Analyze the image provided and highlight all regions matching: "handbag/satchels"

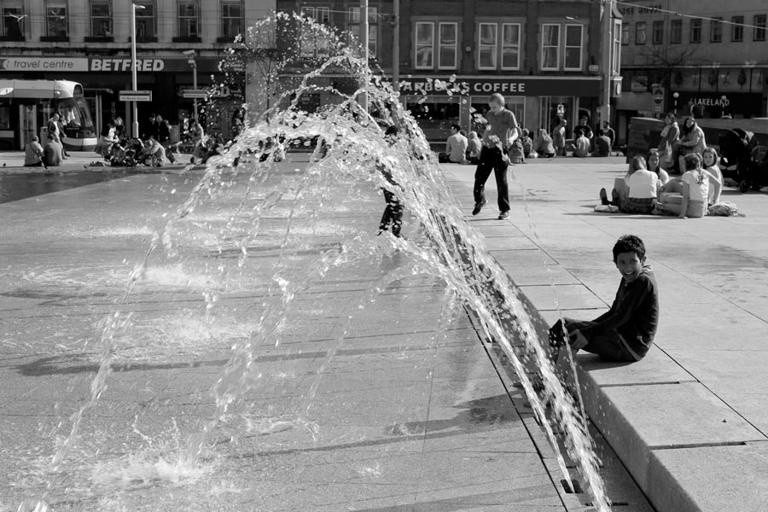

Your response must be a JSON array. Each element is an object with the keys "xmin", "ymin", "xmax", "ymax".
[
  {"xmin": 594, "ymin": 205, "xmax": 618, "ymax": 213},
  {"xmin": 709, "ymin": 201, "xmax": 738, "ymax": 217}
]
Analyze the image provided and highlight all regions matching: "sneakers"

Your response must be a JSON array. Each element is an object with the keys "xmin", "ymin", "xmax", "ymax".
[
  {"xmin": 499, "ymin": 211, "xmax": 510, "ymax": 220},
  {"xmin": 612, "ymin": 188, "xmax": 620, "ymax": 205},
  {"xmin": 600, "ymin": 188, "xmax": 609, "ymax": 205},
  {"xmin": 473, "ymin": 199, "xmax": 487, "ymax": 215}
]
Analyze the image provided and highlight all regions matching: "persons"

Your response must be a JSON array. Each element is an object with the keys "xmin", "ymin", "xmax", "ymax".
[
  {"xmin": 595, "ymin": 110, "xmax": 725, "ymax": 219},
  {"xmin": 472, "ymin": 93, "xmax": 518, "ymax": 222},
  {"xmin": 438, "ymin": 115, "xmax": 594, "ymax": 164},
  {"xmin": 23, "ymin": 112, "xmax": 285, "ymax": 169},
  {"xmin": 514, "ymin": 232, "xmax": 658, "ymax": 407},
  {"xmin": 376, "ymin": 123, "xmax": 416, "ymax": 242}
]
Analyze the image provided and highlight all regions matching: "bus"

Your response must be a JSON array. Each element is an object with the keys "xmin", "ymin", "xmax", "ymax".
[{"xmin": 0, "ymin": 79, "xmax": 97, "ymax": 153}]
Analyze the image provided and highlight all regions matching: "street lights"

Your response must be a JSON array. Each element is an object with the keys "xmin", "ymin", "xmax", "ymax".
[
  {"xmin": 721, "ymin": 95, "xmax": 726, "ymax": 118},
  {"xmin": 185, "ymin": 48, "xmax": 201, "ymax": 126},
  {"xmin": 131, "ymin": 2, "xmax": 148, "ymax": 138},
  {"xmin": 673, "ymin": 92, "xmax": 679, "ymax": 117}
]
